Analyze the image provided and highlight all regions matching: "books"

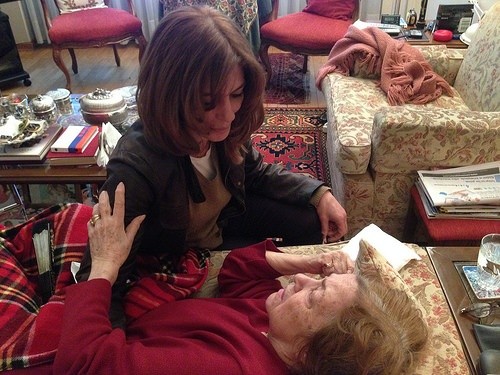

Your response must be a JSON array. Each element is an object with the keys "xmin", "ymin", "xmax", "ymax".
[
  {"xmin": 0, "ymin": 125, "xmax": 103, "ymax": 166},
  {"xmin": 400, "ymin": 29, "xmax": 430, "ymax": 42},
  {"xmin": 415, "ymin": 178, "xmax": 500, "ymax": 222}
]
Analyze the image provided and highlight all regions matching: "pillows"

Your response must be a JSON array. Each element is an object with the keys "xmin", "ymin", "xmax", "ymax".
[
  {"xmin": 56, "ymin": 0, "xmax": 109, "ymax": 14},
  {"xmin": 302, "ymin": 0, "xmax": 357, "ymax": 21}
]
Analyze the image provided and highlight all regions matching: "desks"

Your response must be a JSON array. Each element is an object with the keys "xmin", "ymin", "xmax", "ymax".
[
  {"xmin": 402, "ymin": 186, "xmax": 500, "ymax": 247},
  {"xmin": 426, "ymin": 247, "xmax": 500, "ymax": 375}
]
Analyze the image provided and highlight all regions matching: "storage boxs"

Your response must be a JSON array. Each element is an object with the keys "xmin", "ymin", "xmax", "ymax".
[{"xmin": 436, "ymin": 5, "xmax": 474, "ymax": 34}]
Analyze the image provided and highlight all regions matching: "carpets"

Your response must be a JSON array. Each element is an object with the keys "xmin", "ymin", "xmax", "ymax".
[
  {"xmin": 258, "ymin": 53, "xmax": 311, "ymax": 104},
  {"xmin": 252, "ymin": 106, "xmax": 345, "ymax": 245}
]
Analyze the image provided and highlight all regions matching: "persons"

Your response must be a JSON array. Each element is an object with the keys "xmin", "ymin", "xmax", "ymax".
[
  {"xmin": 65, "ymin": 6, "xmax": 349, "ymax": 268},
  {"xmin": 0, "ymin": 179, "xmax": 427, "ymax": 375}
]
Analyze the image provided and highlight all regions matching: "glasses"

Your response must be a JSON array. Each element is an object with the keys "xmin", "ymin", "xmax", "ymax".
[{"xmin": 460, "ymin": 300, "xmax": 500, "ymax": 318}]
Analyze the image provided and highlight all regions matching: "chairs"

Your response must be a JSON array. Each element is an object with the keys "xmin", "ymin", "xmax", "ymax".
[
  {"xmin": 258, "ymin": 0, "xmax": 360, "ymax": 92},
  {"xmin": 40, "ymin": 0, "xmax": 147, "ymax": 94}
]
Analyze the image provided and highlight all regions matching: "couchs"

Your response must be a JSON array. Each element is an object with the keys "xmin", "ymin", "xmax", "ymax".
[
  {"xmin": 196, "ymin": 244, "xmax": 472, "ymax": 375},
  {"xmin": 320, "ymin": 2, "xmax": 500, "ymax": 247}
]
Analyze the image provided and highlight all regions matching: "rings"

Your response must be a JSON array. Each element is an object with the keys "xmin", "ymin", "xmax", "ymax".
[
  {"xmin": 90, "ymin": 215, "xmax": 100, "ymax": 226},
  {"xmin": 324, "ymin": 262, "xmax": 334, "ymax": 268}
]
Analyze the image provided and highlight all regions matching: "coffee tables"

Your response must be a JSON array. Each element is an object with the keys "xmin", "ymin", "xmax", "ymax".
[{"xmin": 0, "ymin": 95, "xmax": 127, "ymax": 204}]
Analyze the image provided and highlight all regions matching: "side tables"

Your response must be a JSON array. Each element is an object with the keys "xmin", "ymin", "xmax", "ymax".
[{"xmin": 364, "ymin": 18, "xmax": 468, "ymax": 48}]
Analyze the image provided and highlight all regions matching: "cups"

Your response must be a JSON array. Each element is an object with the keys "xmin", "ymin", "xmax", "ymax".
[
  {"xmin": 7, "ymin": 93, "xmax": 28, "ymax": 120},
  {"xmin": 54, "ymin": 88, "xmax": 74, "ymax": 116},
  {"xmin": 476, "ymin": 234, "xmax": 500, "ymax": 292}
]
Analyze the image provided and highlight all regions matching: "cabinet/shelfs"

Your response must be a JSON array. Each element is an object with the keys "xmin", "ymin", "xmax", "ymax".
[{"xmin": 0, "ymin": 11, "xmax": 31, "ymax": 86}]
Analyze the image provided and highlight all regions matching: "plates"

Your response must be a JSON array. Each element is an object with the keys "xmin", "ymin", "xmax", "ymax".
[{"xmin": 0, "ymin": 121, "xmax": 48, "ymax": 146}]
